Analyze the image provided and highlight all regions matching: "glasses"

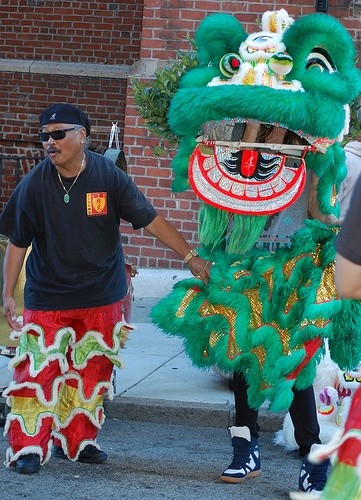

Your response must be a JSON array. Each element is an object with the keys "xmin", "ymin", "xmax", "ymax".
[{"xmin": 38, "ymin": 126, "xmax": 83, "ymax": 142}]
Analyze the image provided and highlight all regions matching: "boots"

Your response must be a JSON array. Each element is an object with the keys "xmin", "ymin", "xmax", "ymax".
[
  {"xmin": 299, "ymin": 457, "xmax": 332, "ymax": 492},
  {"xmin": 221, "ymin": 425, "xmax": 262, "ymax": 483}
]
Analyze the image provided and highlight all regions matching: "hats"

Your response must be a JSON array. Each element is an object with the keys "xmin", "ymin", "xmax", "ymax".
[{"xmin": 39, "ymin": 104, "xmax": 90, "ymax": 137}]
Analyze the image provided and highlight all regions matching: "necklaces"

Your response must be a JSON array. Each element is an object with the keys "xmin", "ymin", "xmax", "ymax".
[{"xmin": 49, "ymin": 151, "xmax": 85, "ymax": 204}]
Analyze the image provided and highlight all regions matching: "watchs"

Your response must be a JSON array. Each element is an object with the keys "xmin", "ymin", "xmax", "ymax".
[{"xmin": 183, "ymin": 249, "xmax": 198, "ymax": 263}]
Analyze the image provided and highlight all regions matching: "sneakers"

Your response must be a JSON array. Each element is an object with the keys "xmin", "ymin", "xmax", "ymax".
[
  {"xmin": 16, "ymin": 453, "xmax": 40, "ymax": 473},
  {"xmin": 54, "ymin": 443, "xmax": 107, "ymax": 463}
]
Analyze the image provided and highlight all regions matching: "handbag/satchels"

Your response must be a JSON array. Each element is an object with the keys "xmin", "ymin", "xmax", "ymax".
[{"xmin": 103, "ymin": 122, "xmax": 128, "ymax": 175}]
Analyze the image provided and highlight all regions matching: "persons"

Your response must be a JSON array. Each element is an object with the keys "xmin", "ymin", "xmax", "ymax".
[
  {"xmin": 1, "ymin": 104, "xmax": 215, "ymax": 468},
  {"xmin": 220, "ymin": 120, "xmax": 331, "ymax": 492},
  {"xmin": 333, "ymin": 170, "xmax": 361, "ymax": 300}
]
